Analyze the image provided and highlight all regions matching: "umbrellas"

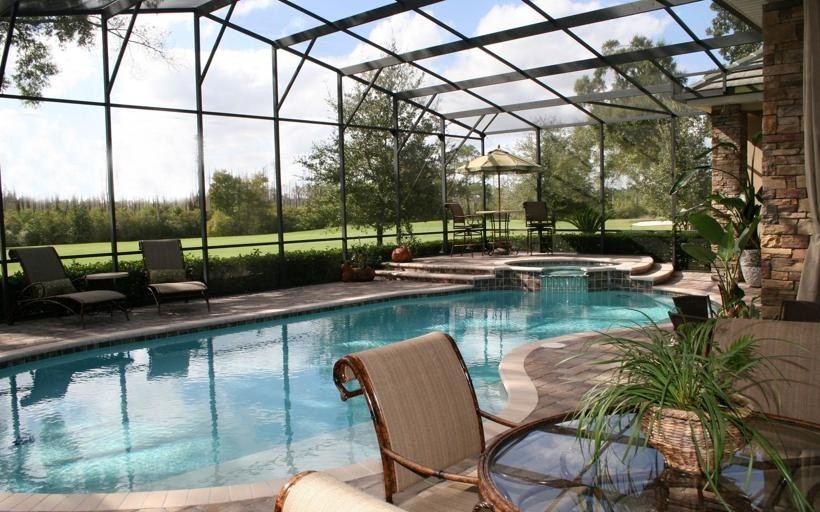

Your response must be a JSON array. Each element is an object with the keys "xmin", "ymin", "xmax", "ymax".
[{"xmin": 456, "ymin": 144, "xmax": 542, "ymax": 236}]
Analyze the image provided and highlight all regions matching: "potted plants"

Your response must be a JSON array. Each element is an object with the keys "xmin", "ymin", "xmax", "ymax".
[
  {"xmin": 667, "ymin": 128, "xmax": 764, "ymax": 287},
  {"xmin": 342, "ymin": 242, "xmax": 383, "ymax": 282},
  {"xmin": 568, "ymin": 304, "xmax": 820, "ymax": 512}
]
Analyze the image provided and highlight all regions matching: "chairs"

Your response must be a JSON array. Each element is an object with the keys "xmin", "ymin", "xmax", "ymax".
[
  {"xmin": 522, "ymin": 201, "xmax": 556, "ymax": 256},
  {"xmin": 8, "ymin": 238, "xmax": 212, "ymax": 329},
  {"xmin": 275, "ymin": 466, "xmax": 414, "ymax": 512},
  {"xmin": 330, "ymin": 334, "xmax": 523, "ymax": 512},
  {"xmin": 445, "ymin": 202, "xmax": 484, "ymax": 258}
]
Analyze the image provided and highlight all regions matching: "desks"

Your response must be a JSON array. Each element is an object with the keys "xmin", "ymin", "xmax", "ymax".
[
  {"xmin": 476, "ymin": 402, "xmax": 818, "ymax": 512},
  {"xmin": 475, "ymin": 209, "xmax": 524, "ymax": 256}
]
